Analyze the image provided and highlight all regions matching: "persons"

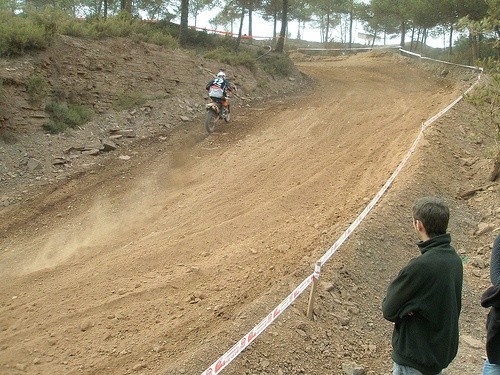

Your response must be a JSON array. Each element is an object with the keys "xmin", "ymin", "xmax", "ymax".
[
  {"xmin": 380, "ymin": 196, "xmax": 464, "ymax": 375},
  {"xmin": 205, "ymin": 71, "xmax": 236, "ymax": 120},
  {"xmin": 480, "ymin": 234, "xmax": 499, "ymax": 375}
]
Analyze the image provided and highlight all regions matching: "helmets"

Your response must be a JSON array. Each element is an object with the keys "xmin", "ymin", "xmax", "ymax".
[{"xmin": 216, "ymin": 71, "xmax": 226, "ymax": 77}]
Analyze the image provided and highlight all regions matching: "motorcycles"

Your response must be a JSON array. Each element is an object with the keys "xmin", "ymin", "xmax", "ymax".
[{"xmin": 205, "ymin": 87, "xmax": 231, "ymax": 133}]
